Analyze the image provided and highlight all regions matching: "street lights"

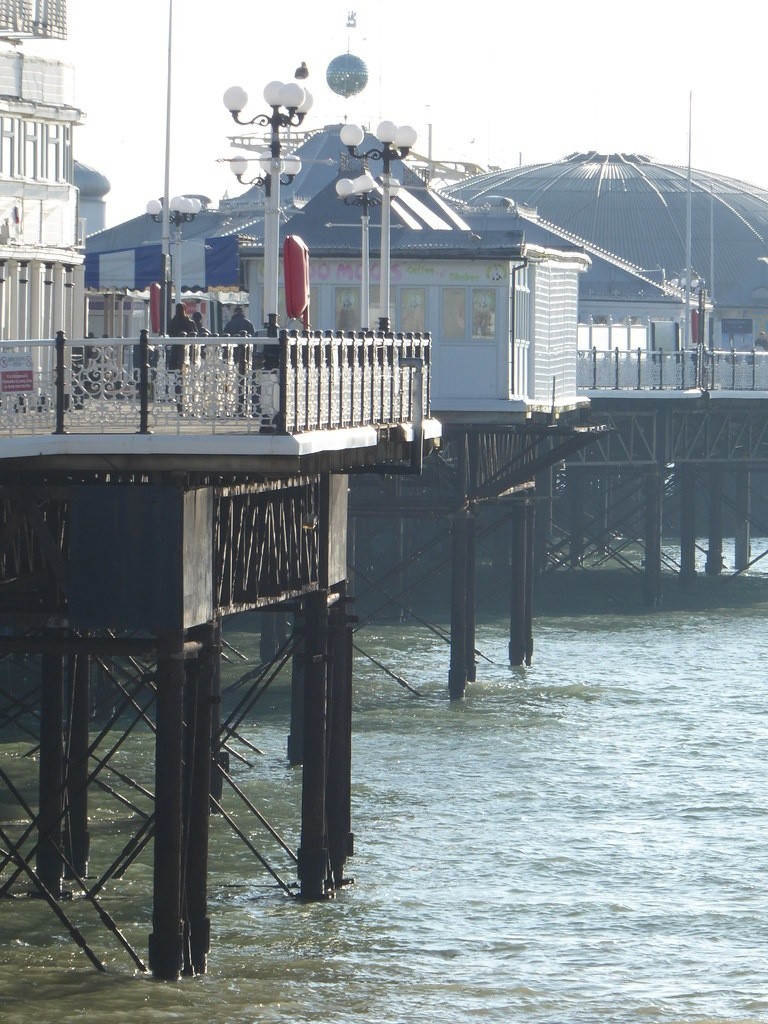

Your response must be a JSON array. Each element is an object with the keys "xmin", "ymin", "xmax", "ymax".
[
  {"xmin": 336, "ymin": 175, "xmax": 401, "ymax": 332},
  {"xmin": 214, "ymin": 80, "xmax": 313, "ymax": 434},
  {"xmin": 146, "ymin": 196, "xmax": 202, "ymax": 305},
  {"xmin": 670, "ymin": 277, "xmax": 699, "ymax": 361},
  {"xmin": 340, "ymin": 120, "xmax": 418, "ymax": 332},
  {"xmin": 231, "ymin": 152, "xmax": 302, "ymax": 336}
]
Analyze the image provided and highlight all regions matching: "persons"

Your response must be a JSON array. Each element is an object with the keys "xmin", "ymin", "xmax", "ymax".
[
  {"xmin": 192, "ymin": 306, "xmax": 255, "ymax": 365},
  {"xmin": 168, "ymin": 303, "xmax": 197, "ymax": 413},
  {"xmin": 755, "ymin": 332, "xmax": 767, "ymax": 350}
]
